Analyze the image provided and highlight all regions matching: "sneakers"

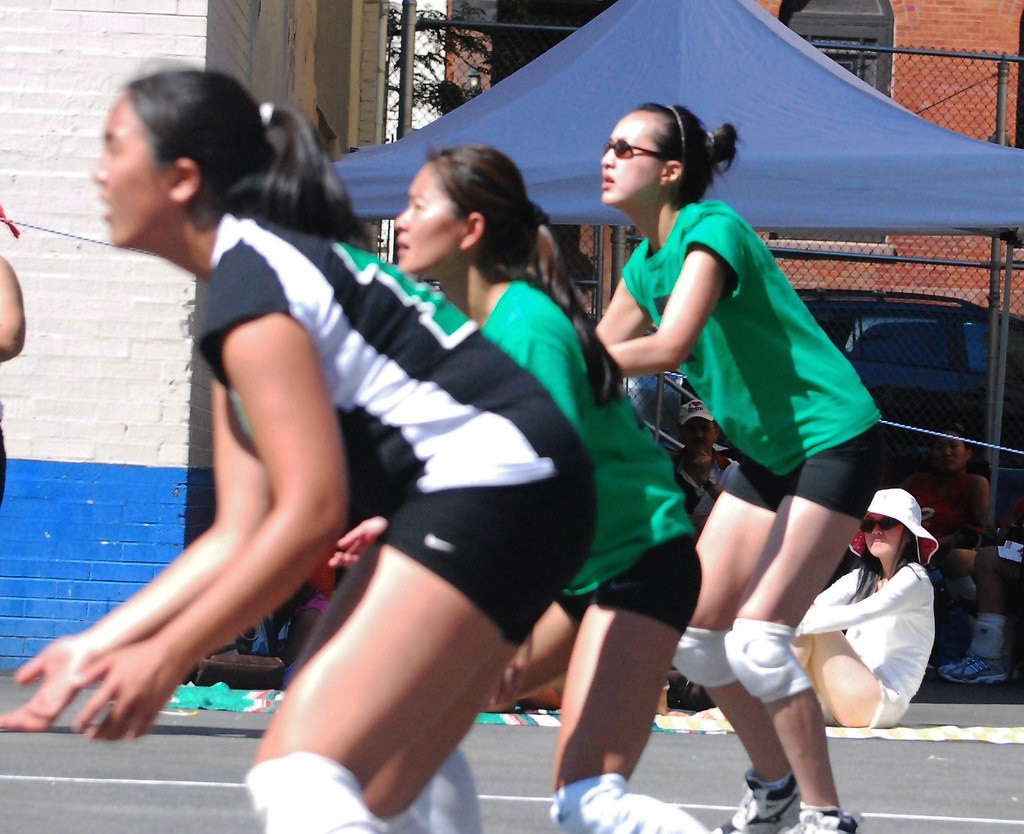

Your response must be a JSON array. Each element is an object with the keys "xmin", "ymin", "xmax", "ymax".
[
  {"xmin": 712, "ymin": 772, "xmax": 800, "ymax": 834},
  {"xmin": 783, "ymin": 802, "xmax": 858, "ymax": 834},
  {"xmin": 938, "ymin": 655, "xmax": 1009, "ymax": 684}
]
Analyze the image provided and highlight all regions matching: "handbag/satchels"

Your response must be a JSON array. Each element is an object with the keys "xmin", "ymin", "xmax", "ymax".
[{"xmin": 667, "ymin": 672, "xmax": 708, "ymax": 712}]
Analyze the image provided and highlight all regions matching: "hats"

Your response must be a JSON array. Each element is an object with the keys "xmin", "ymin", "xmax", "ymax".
[
  {"xmin": 848, "ymin": 488, "xmax": 939, "ymax": 566},
  {"xmin": 679, "ymin": 399, "xmax": 714, "ymax": 425}
]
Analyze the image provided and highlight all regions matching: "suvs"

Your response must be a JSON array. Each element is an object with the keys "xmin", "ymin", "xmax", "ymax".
[{"xmin": 793, "ymin": 288, "xmax": 1024, "ymax": 470}]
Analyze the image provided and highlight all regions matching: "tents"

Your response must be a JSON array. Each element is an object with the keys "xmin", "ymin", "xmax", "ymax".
[{"xmin": 330, "ymin": 0, "xmax": 1024, "ymax": 521}]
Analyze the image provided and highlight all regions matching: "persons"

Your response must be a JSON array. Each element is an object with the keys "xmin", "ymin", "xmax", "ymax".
[
  {"xmin": 0, "ymin": 255, "xmax": 26, "ymax": 506},
  {"xmin": 938, "ymin": 499, "xmax": 1024, "ymax": 683},
  {"xmin": 790, "ymin": 487, "xmax": 937, "ymax": 729},
  {"xmin": 897, "ymin": 425, "xmax": 990, "ymax": 595},
  {"xmin": 0, "ymin": 69, "xmax": 598, "ymax": 834},
  {"xmin": 399, "ymin": 143, "xmax": 713, "ymax": 834},
  {"xmin": 664, "ymin": 399, "xmax": 732, "ymax": 515},
  {"xmin": 599, "ymin": 103, "xmax": 882, "ymax": 834}
]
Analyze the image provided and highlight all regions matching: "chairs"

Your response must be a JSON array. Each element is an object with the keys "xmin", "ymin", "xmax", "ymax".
[{"xmin": 995, "ymin": 465, "xmax": 1024, "ymax": 530}]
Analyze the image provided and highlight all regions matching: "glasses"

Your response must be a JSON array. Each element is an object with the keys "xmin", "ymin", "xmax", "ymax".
[
  {"xmin": 603, "ymin": 139, "xmax": 670, "ymax": 161},
  {"xmin": 860, "ymin": 517, "xmax": 901, "ymax": 532}
]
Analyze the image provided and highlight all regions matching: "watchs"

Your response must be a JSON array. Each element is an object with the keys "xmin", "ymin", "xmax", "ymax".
[{"xmin": 704, "ymin": 479, "xmax": 718, "ymax": 490}]
